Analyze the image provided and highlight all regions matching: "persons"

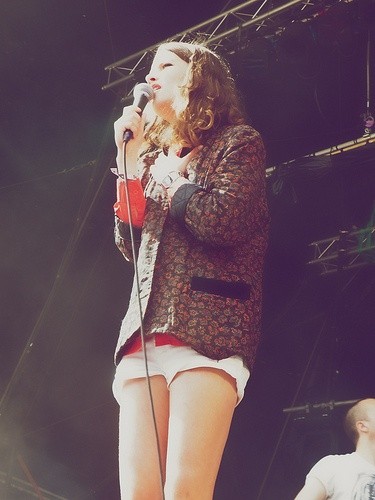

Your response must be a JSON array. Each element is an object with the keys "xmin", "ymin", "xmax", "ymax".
[
  {"xmin": 294, "ymin": 396, "xmax": 374, "ymax": 500},
  {"xmin": 110, "ymin": 40, "xmax": 270, "ymax": 500}
]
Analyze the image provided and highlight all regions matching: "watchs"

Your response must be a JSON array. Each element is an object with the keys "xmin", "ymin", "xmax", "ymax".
[{"xmin": 162, "ymin": 170, "xmax": 184, "ymax": 189}]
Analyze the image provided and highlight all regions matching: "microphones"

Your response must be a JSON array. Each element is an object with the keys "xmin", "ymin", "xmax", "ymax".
[{"xmin": 123, "ymin": 83, "xmax": 154, "ymax": 142}]
